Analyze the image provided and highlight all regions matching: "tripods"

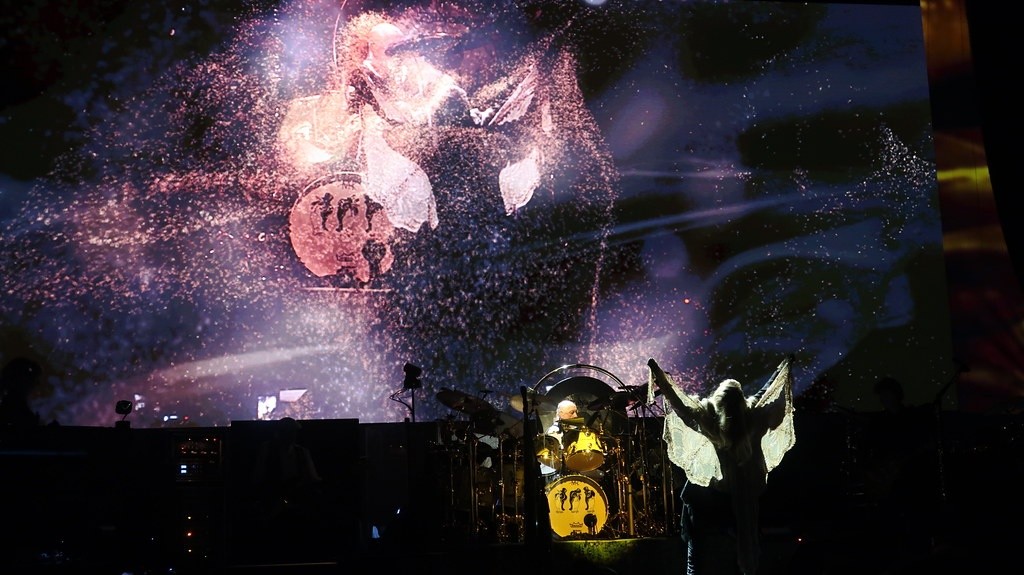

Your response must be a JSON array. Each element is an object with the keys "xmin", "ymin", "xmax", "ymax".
[{"xmin": 608, "ymin": 401, "xmax": 665, "ymax": 539}]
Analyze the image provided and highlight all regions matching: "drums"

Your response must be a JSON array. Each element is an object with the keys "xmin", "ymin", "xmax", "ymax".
[
  {"xmin": 533, "ymin": 434, "xmax": 561, "ymax": 477},
  {"xmin": 542, "ymin": 473, "xmax": 611, "ymax": 542},
  {"xmin": 561, "ymin": 427, "xmax": 606, "ymax": 474}
]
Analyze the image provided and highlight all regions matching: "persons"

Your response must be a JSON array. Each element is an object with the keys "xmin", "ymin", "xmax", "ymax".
[
  {"xmin": 646, "ymin": 357, "xmax": 798, "ymax": 575},
  {"xmin": 541, "ymin": 401, "xmax": 586, "ymax": 450}
]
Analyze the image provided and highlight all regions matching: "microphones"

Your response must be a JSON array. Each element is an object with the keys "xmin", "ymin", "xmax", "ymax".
[{"xmin": 481, "ymin": 390, "xmax": 493, "ymax": 393}]
